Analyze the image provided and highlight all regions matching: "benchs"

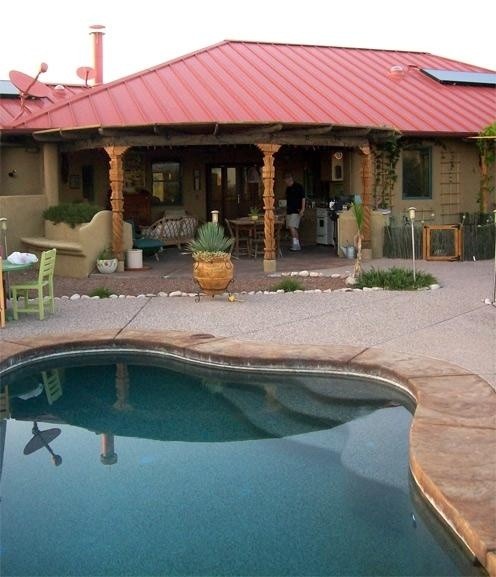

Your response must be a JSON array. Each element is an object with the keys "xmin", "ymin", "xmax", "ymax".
[{"xmin": 138, "ymin": 215, "xmax": 200, "ymax": 262}]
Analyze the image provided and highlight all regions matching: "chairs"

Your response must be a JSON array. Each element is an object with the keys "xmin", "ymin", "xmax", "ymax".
[
  {"xmin": 225, "ymin": 214, "xmax": 285, "ymax": 258},
  {"xmin": 11, "ymin": 247, "xmax": 57, "ymax": 321}
]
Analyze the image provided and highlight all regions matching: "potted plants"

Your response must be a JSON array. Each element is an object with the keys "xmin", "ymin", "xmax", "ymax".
[
  {"xmin": 181, "ymin": 222, "xmax": 238, "ymax": 295},
  {"xmin": 248, "ymin": 206, "xmax": 259, "ymax": 220},
  {"xmin": 94, "ymin": 246, "xmax": 118, "ymax": 273}
]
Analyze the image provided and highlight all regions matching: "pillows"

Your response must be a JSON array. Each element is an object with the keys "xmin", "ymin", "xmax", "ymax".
[{"xmin": 151, "ymin": 218, "xmax": 196, "ymax": 239}]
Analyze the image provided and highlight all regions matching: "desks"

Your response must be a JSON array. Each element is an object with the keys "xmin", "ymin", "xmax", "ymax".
[{"xmin": 1, "ymin": 259, "xmax": 34, "ymax": 323}]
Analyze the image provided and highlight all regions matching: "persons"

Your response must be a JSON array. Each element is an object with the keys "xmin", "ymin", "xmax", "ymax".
[{"xmin": 281, "ymin": 172, "xmax": 306, "ymax": 251}]
